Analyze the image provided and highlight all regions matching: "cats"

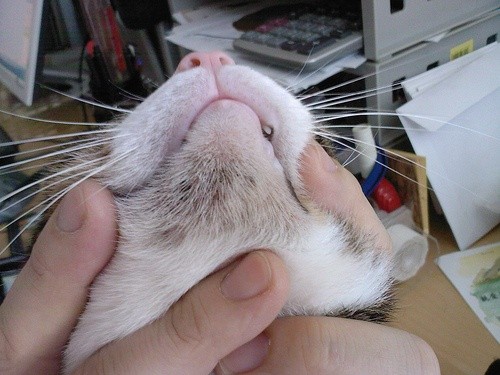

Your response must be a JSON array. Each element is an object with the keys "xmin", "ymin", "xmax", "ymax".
[{"xmin": 2, "ymin": 45, "xmax": 498, "ymax": 370}]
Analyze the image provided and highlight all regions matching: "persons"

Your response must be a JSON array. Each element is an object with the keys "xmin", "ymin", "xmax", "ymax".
[{"xmin": 1, "ymin": 178, "xmax": 442, "ymax": 373}]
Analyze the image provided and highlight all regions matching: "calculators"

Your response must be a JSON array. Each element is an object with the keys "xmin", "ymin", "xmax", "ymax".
[{"xmin": 232, "ymin": 2, "xmax": 364, "ymax": 72}]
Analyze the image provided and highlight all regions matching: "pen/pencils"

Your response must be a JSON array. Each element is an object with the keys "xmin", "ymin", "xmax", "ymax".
[{"xmin": 83, "ymin": 35, "xmax": 142, "ymax": 94}]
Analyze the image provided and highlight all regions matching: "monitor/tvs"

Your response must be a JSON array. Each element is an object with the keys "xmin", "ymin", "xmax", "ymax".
[{"xmin": 0, "ymin": 0, "xmax": 45, "ymax": 106}]
